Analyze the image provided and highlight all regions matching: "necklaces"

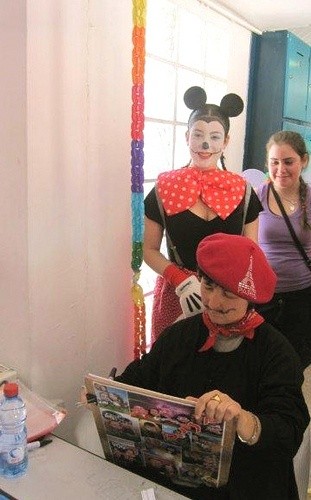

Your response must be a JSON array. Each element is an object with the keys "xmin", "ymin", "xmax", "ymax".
[{"xmin": 280, "ymin": 195, "xmax": 298, "ymax": 212}]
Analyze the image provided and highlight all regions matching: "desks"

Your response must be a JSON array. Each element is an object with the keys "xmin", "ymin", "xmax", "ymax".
[{"xmin": 0, "ymin": 433, "xmax": 191, "ymax": 500}]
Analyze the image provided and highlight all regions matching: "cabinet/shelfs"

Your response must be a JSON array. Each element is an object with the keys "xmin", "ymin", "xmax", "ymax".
[{"xmin": 247, "ymin": 29, "xmax": 311, "ymax": 173}]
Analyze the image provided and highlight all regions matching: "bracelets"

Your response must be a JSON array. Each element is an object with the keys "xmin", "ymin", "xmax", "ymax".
[{"xmin": 238, "ymin": 411, "xmax": 258, "ymax": 444}]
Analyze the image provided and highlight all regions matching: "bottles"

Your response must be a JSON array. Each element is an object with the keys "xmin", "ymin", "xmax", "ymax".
[{"xmin": 0, "ymin": 384, "xmax": 28, "ymax": 478}]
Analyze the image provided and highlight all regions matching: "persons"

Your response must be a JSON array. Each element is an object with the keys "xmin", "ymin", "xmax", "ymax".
[
  {"xmin": 143, "ymin": 105, "xmax": 265, "ymax": 349},
  {"xmin": 95, "ymin": 383, "xmax": 223, "ymax": 489},
  {"xmin": 81, "ymin": 233, "xmax": 311, "ymax": 500},
  {"xmin": 253, "ymin": 131, "xmax": 311, "ymax": 364}
]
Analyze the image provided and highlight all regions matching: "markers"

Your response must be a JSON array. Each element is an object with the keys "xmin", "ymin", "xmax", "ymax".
[
  {"xmin": 107, "ymin": 367, "xmax": 116, "ymax": 381},
  {"xmin": 27, "ymin": 439, "xmax": 53, "ymax": 452}
]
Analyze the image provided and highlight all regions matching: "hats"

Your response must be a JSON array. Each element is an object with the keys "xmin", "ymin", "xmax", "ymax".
[{"xmin": 196, "ymin": 233, "xmax": 278, "ymax": 304}]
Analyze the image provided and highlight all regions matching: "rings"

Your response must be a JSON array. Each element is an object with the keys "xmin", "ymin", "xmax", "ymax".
[{"xmin": 211, "ymin": 395, "xmax": 221, "ymax": 402}]
